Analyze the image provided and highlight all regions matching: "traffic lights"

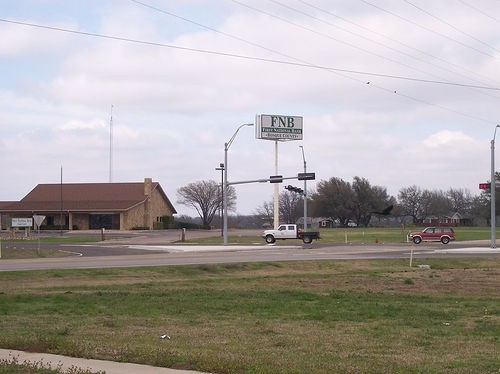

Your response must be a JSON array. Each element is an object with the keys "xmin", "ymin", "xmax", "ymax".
[
  {"xmin": 288, "ymin": 185, "xmax": 303, "ymax": 194},
  {"xmin": 479, "ymin": 184, "xmax": 490, "ymax": 189}
]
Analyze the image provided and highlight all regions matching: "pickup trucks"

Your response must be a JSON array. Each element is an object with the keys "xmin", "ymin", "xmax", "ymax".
[{"xmin": 261, "ymin": 224, "xmax": 320, "ymax": 244}]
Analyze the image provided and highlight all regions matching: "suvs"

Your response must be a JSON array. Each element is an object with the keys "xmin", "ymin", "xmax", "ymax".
[{"xmin": 408, "ymin": 226, "xmax": 456, "ymax": 244}]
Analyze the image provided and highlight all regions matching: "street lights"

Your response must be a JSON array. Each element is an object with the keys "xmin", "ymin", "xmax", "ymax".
[
  {"xmin": 298, "ymin": 145, "xmax": 308, "ymax": 231},
  {"xmin": 490, "ymin": 124, "xmax": 500, "ymax": 248},
  {"xmin": 223, "ymin": 123, "xmax": 254, "ymax": 245}
]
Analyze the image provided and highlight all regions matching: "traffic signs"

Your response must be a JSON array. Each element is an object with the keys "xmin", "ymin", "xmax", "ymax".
[{"xmin": 12, "ymin": 218, "xmax": 32, "ymax": 227}]
